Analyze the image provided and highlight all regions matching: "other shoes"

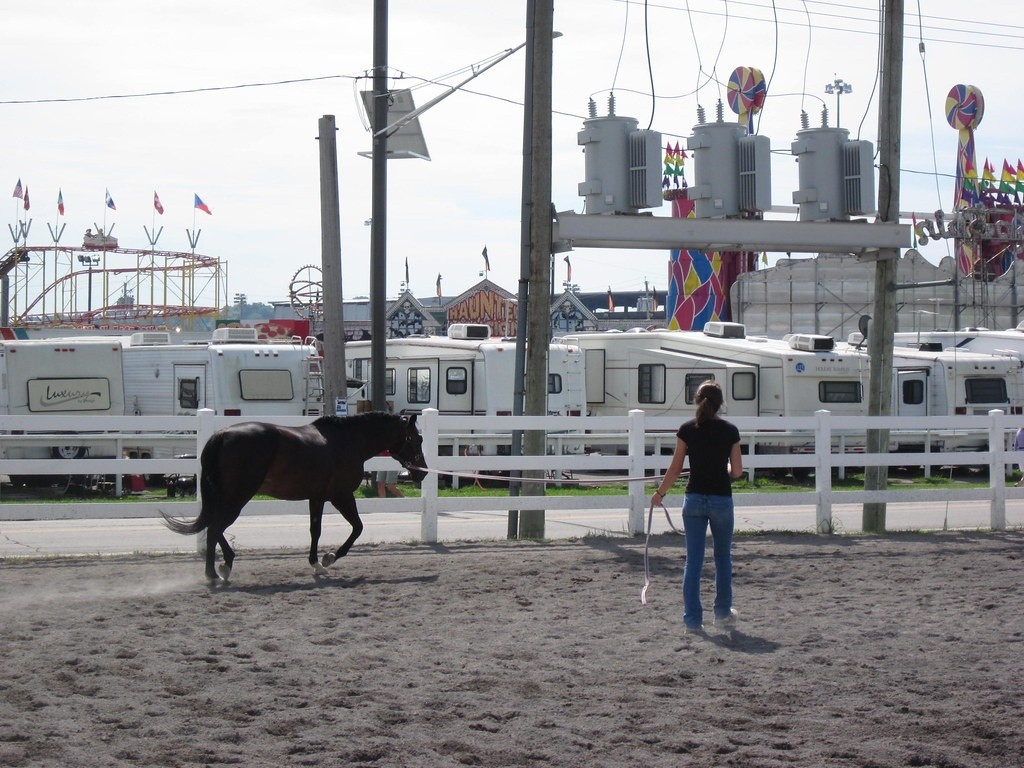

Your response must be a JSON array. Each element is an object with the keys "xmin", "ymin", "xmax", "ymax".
[
  {"xmin": 1014, "ymin": 483, "xmax": 1021, "ymax": 487},
  {"xmin": 684, "ymin": 625, "xmax": 704, "ymax": 636},
  {"xmin": 713, "ymin": 608, "xmax": 739, "ymax": 627}
]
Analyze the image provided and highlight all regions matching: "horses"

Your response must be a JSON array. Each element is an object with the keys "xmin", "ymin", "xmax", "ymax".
[{"xmin": 159, "ymin": 410, "xmax": 428, "ymax": 584}]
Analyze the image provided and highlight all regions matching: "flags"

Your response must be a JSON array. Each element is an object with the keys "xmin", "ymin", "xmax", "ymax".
[
  {"xmin": 57, "ymin": 189, "xmax": 64, "ymax": 215},
  {"xmin": 12, "ymin": 178, "xmax": 23, "ymax": 199},
  {"xmin": 105, "ymin": 190, "xmax": 116, "ymax": 209},
  {"xmin": 24, "ymin": 185, "xmax": 30, "ymax": 210},
  {"xmin": 195, "ymin": 195, "xmax": 213, "ymax": 215},
  {"xmin": 154, "ymin": 193, "xmax": 164, "ymax": 215}
]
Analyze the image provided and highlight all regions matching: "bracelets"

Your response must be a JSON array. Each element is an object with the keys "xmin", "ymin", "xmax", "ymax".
[{"xmin": 656, "ymin": 490, "xmax": 666, "ymax": 499}]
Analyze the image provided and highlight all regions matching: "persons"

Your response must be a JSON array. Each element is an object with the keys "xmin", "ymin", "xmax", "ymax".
[{"xmin": 652, "ymin": 380, "xmax": 742, "ymax": 633}]
